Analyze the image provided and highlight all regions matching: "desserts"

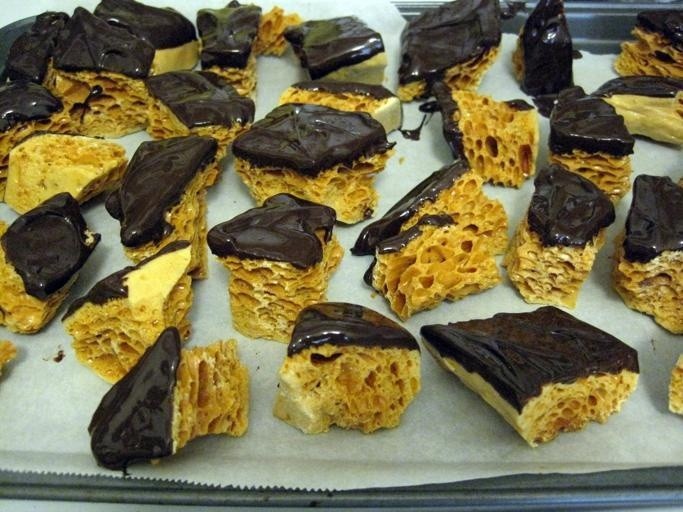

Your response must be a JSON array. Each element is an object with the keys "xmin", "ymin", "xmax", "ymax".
[{"xmin": 0, "ymin": 0, "xmax": 683, "ymax": 471}]
